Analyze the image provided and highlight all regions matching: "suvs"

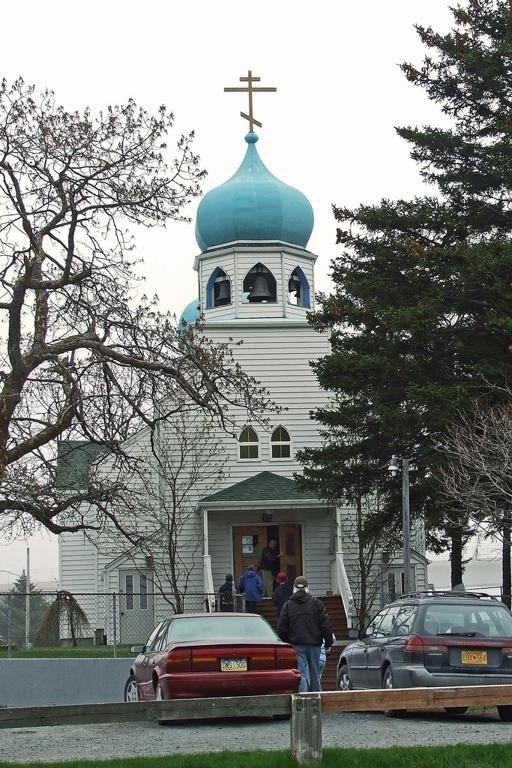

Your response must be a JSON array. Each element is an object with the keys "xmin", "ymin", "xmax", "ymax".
[{"xmin": 334, "ymin": 589, "xmax": 512, "ymax": 720}]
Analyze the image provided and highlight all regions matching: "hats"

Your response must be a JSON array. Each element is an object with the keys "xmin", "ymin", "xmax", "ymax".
[
  {"xmin": 277, "ymin": 572, "xmax": 288, "ymax": 582},
  {"xmin": 294, "ymin": 576, "xmax": 308, "ymax": 588}
]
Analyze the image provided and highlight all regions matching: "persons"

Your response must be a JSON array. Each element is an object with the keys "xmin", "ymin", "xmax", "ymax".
[
  {"xmin": 260, "ymin": 539, "xmax": 283, "ymax": 598},
  {"xmin": 272, "ymin": 572, "xmax": 293, "ymax": 621},
  {"xmin": 278, "ymin": 576, "xmax": 336, "ymax": 692},
  {"xmin": 237, "ymin": 564, "xmax": 264, "ymax": 613},
  {"xmin": 219, "ymin": 574, "xmax": 237, "ymax": 612}
]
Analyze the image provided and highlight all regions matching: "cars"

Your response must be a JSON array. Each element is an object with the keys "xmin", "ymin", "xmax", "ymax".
[
  {"xmin": 0, "ymin": 635, "xmax": 17, "ymax": 652},
  {"xmin": 120, "ymin": 610, "xmax": 303, "ymax": 725}
]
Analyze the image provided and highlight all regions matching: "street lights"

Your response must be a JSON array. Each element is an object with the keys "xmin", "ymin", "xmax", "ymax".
[{"xmin": 386, "ymin": 453, "xmax": 414, "ymax": 593}]
